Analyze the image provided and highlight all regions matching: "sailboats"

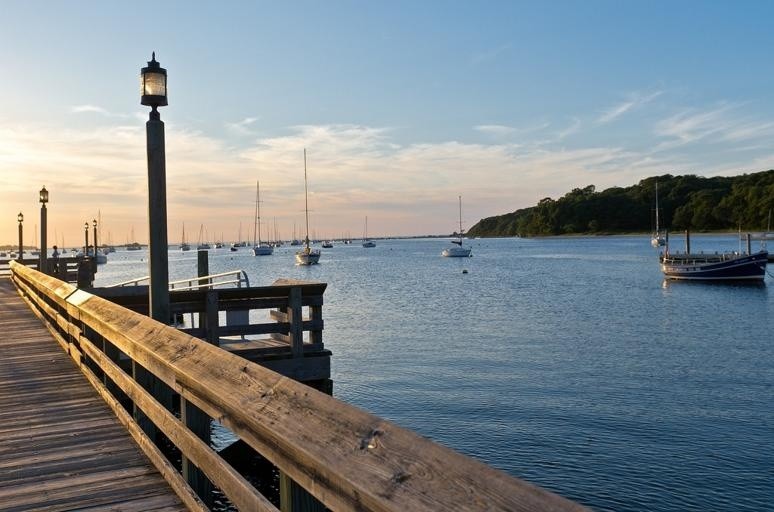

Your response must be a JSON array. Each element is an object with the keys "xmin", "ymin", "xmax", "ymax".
[
  {"xmin": 179, "ymin": 222, "xmax": 190, "ymax": 252},
  {"xmin": 441, "ymin": 196, "xmax": 471, "ymax": 257},
  {"xmin": 741, "ymin": 208, "xmax": 774, "ymax": 240},
  {"xmin": 251, "ymin": 181, "xmax": 273, "ymax": 256},
  {"xmin": 196, "ymin": 224, "xmax": 210, "ymax": 250},
  {"xmin": 294, "ymin": 148, "xmax": 320, "ymax": 265},
  {"xmin": 651, "ymin": 182, "xmax": 665, "ymax": 247},
  {"xmin": 361, "ymin": 216, "xmax": 377, "ymax": 248}
]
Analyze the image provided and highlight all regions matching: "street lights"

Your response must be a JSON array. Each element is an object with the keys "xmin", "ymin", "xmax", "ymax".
[
  {"xmin": 139, "ymin": 51, "xmax": 170, "ymax": 323},
  {"xmin": 84, "ymin": 222, "xmax": 89, "ymax": 255},
  {"xmin": 17, "ymin": 212, "xmax": 23, "ymax": 264},
  {"xmin": 39, "ymin": 185, "xmax": 48, "ymax": 274},
  {"xmin": 92, "ymin": 218, "xmax": 97, "ymax": 256}
]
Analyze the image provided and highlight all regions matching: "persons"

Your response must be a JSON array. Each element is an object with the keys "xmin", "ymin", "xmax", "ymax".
[{"xmin": 52, "ymin": 246, "xmax": 59, "ymax": 272}]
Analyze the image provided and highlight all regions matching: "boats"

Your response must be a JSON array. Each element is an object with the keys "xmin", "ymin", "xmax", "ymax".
[
  {"xmin": 660, "ymin": 229, "xmax": 774, "ymax": 285},
  {"xmin": 232, "ymin": 241, "xmax": 250, "ymax": 247},
  {"xmin": 230, "ymin": 247, "xmax": 238, "ymax": 252},
  {"xmin": 126, "ymin": 246, "xmax": 141, "ymax": 250},
  {"xmin": 214, "ymin": 240, "xmax": 222, "ymax": 249},
  {"xmin": 0, "ymin": 240, "xmax": 117, "ymax": 265},
  {"xmin": 342, "ymin": 232, "xmax": 353, "ymax": 245},
  {"xmin": 322, "ymin": 241, "xmax": 333, "ymax": 249},
  {"xmin": 275, "ymin": 238, "xmax": 320, "ymax": 247}
]
